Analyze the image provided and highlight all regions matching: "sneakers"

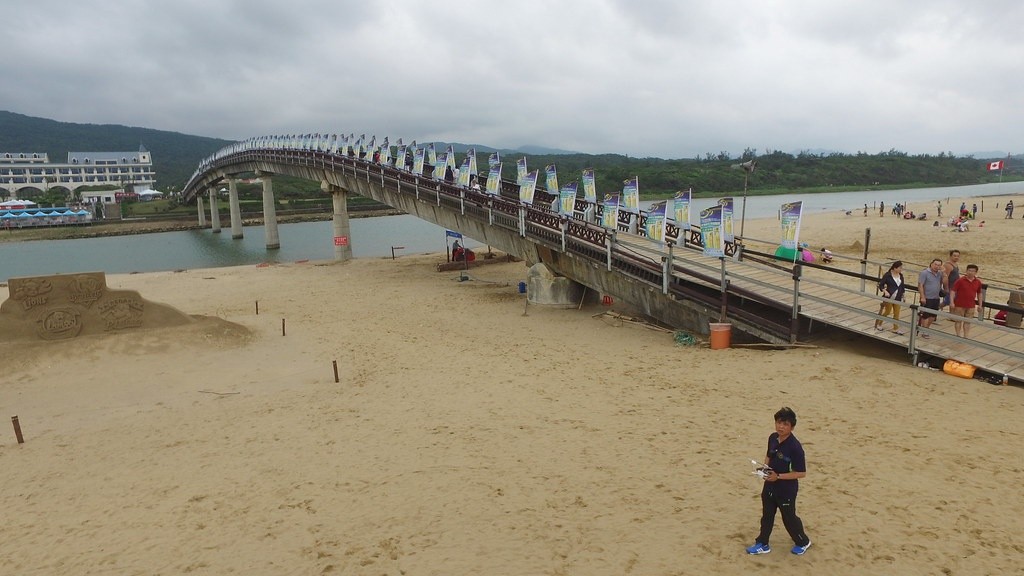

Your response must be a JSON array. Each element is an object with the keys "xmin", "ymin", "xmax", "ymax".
[
  {"xmin": 746, "ymin": 541, "xmax": 771, "ymax": 555},
  {"xmin": 791, "ymin": 540, "xmax": 811, "ymax": 555}
]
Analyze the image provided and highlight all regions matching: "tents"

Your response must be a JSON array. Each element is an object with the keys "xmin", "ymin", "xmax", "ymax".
[{"xmin": 0, "ymin": 209, "xmax": 90, "ymax": 222}]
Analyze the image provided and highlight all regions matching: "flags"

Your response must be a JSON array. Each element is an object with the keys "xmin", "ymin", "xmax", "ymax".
[{"xmin": 987, "ymin": 160, "xmax": 1004, "ymax": 171}]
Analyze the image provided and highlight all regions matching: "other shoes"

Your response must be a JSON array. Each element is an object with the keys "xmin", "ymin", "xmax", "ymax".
[
  {"xmin": 922, "ymin": 332, "xmax": 930, "ymax": 339},
  {"xmin": 877, "ymin": 325, "xmax": 883, "ymax": 331},
  {"xmin": 893, "ymin": 328, "xmax": 903, "ymax": 334}
]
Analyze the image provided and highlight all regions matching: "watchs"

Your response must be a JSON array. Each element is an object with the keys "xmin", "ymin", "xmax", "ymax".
[{"xmin": 777, "ymin": 473, "xmax": 779, "ymax": 480}]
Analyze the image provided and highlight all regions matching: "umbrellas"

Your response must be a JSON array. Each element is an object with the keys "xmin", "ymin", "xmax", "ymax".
[
  {"xmin": 874, "ymin": 296, "xmax": 887, "ymax": 331},
  {"xmin": 915, "ymin": 300, "xmax": 924, "ymax": 336}
]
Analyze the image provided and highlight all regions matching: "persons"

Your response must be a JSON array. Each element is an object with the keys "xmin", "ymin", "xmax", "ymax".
[
  {"xmin": 746, "ymin": 407, "xmax": 811, "ymax": 555},
  {"xmin": 876, "ymin": 261, "xmax": 906, "ymax": 334},
  {"xmin": 845, "ymin": 200, "xmax": 1024, "ymax": 233},
  {"xmin": 452, "ymin": 240, "xmax": 461, "ymax": 261},
  {"xmin": 917, "ymin": 249, "xmax": 1009, "ymax": 338},
  {"xmin": 796, "ymin": 242, "xmax": 833, "ymax": 263},
  {"xmin": 375, "ymin": 151, "xmax": 485, "ymax": 194}
]
